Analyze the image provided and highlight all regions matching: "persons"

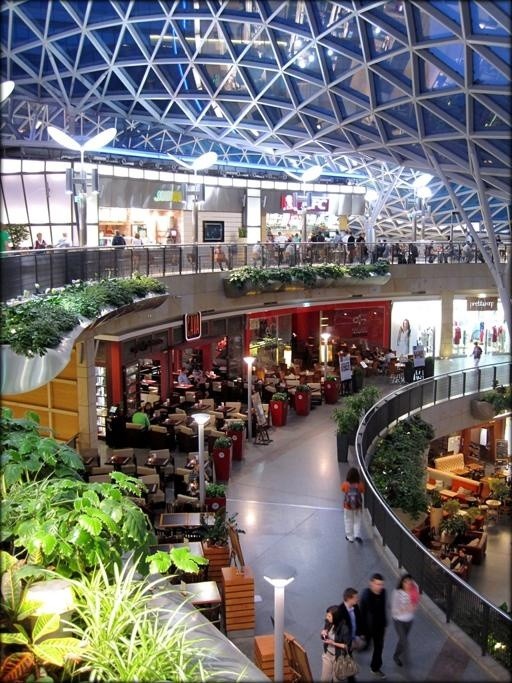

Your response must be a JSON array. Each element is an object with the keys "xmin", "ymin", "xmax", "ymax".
[
  {"xmin": 0, "ymin": 221, "xmax": 13, "ymax": 258},
  {"xmin": 131, "ymin": 402, "xmax": 198, "ymax": 437},
  {"xmin": 469, "ymin": 341, "xmax": 482, "ymax": 376},
  {"xmin": 388, "ymin": 574, "xmax": 424, "ymax": 671},
  {"xmin": 338, "ymin": 465, "xmax": 367, "ymax": 546},
  {"xmin": 34, "ymin": 231, "xmax": 47, "ymax": 254},
  {"xmin": 383, "ymin": 348, "xmax": 396, "ymax": 376},
  {"xmin": 56, "ymin": 229, "xmax": 71, "ymax": 251},
  {"xmin": 178, "ymin": 365, "xmax": 192, "ymax": 386},
  {"xmin": 396, "ymin": 317, "xmax": 412, "ymax": 356},
  {"xmin": 317, "ymin": 605, "xmax": 351, "ymax": 682},
  {"xmin": 111, "ymin": 230, "xmax": 127, "ymax": 249},
  {"xmin": 131, "ymin": 232, "xmax": 145, "ymax": 249},
  {"xmin": 454, "ymin": 319, "xmax": 506, "ymax": 351},
  {"xmin": 337, "ymin": 587, "xmax": 367, "ymax": 682},
  {"xmin": 214, "ymin": 229, "xmax": 507, "ymax": 271},
  {"xmin": 358, "ymin": 573, "xmax": 388, "ymax": 679}
]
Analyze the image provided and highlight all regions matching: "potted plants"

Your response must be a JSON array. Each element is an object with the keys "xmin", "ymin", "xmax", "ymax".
[
  {"xmin": 330, "ymin": 384, "xmax": 378, "ymax": 462},
  {"xmin": 323, "ymin": 374, "xmax": 337, "ymax": 404}
]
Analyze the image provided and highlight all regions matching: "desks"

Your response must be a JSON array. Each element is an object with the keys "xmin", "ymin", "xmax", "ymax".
[{"xmin": 142, "ymin": 512, "xmax": 228, "ymax": 614}]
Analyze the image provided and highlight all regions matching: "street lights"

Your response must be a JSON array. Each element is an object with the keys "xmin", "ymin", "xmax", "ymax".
[
  {"xmin": 45, "ymin": 125, "xmax": 118, "ymax": 279},
  {"xmin": 281, "ymin": 165, "xmax": 322, "ymax": 263},
  {"xmin": 189, "ymin": 412, "xmax": 214, "ymax": 506},
  {"xmin": 263, "ymin": 563, "xmax": 298, "ymax": 682},
  {"xmin": 167, "ymin": 150, "xmax": 218, "ymax": 273},
  {"xmin": 416, "ymin": 185, "xmax": 431, "ymax": 252},
  {"xmin": 243, "ymin": 356, "xmax": 257, "ymax": 442},
  {"xmin": 320, "ymin": 332, "xmax": 332, "ymax": 380}
]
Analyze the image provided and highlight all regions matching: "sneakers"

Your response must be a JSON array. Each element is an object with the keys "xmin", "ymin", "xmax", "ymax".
[
  {"xmin": 370, "ymin": 669, "xmax": 386, "ymax": 679},
  {"xmin": 356, "ymin": 537, "xmax": 363, "ymax": 543},
  {"xmin": 393, "ymin": 656, "xmax": 403, "ymax": 667},
  {"xmin": 345, "ymin": 536, "xmax": 353, "ymax": 543}
]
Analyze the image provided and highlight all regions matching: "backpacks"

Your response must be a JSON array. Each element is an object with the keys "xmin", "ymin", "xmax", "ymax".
[{"xmin": 343, "ymin": 483, "xmax": 362, "ymax": 510}]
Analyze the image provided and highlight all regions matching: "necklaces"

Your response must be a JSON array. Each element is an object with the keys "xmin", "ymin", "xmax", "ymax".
[{"xmin": 370, "ymin": 346, "xmax": 383, "ymax": 376}]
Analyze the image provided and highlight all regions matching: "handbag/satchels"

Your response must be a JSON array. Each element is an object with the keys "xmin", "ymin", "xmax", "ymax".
[{"xmin": 335, "ymin": 654, "xmax": 361, "ymax": 681}]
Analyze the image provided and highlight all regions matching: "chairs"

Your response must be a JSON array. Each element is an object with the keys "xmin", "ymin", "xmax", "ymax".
[
  {"xmin": 412, "ymin": 453, "xmax": 510, "ymax": 573},
  {"xmin": 65, "ymin": 369, "xmax": 320, "ymax": 517}
]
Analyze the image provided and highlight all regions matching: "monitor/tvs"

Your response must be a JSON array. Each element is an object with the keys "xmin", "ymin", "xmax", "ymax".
[{"xmin": 185, "ymin": 312, "xmax": 202, "ymax": 342}]
